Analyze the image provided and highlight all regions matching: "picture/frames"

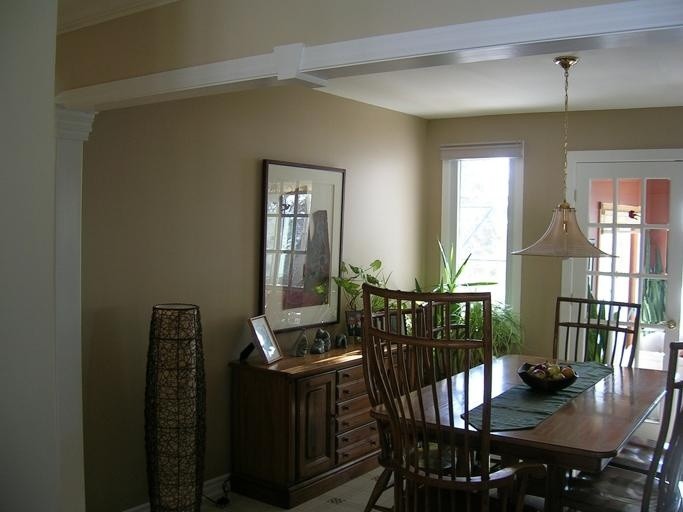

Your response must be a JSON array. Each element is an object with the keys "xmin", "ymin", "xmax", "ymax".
[
  {"xmin": 236, "ymin": 314, "xmax": 284, "ymax": 367},
  {"xmin": 259, "ymin": 158, "xmax": 347, "ymax": 334}
]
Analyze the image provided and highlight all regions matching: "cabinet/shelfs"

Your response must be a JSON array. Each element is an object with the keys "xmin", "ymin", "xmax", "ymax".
[{"xmin": 228, "ymin": 341, "xmax": 491, "ymax": 506}]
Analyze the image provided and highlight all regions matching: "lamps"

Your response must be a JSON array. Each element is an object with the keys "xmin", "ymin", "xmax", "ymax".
[
  {"xmin": 143, "ymin": 300, "xmax": 210, "ymax": 510},
  {"xmin": 507, "ymin": 53, "xmax": 620, "ymax": 265}
]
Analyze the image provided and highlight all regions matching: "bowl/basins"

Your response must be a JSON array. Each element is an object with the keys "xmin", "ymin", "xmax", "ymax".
[{"xmin": 517, "ymin": 362, "xmax": 580, "ymax": 392}]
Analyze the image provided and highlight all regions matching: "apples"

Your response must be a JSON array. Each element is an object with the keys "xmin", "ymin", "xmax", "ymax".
[{"xmin": 526, "ymin": 363, "xmax": 574, "ymax": 382}]
